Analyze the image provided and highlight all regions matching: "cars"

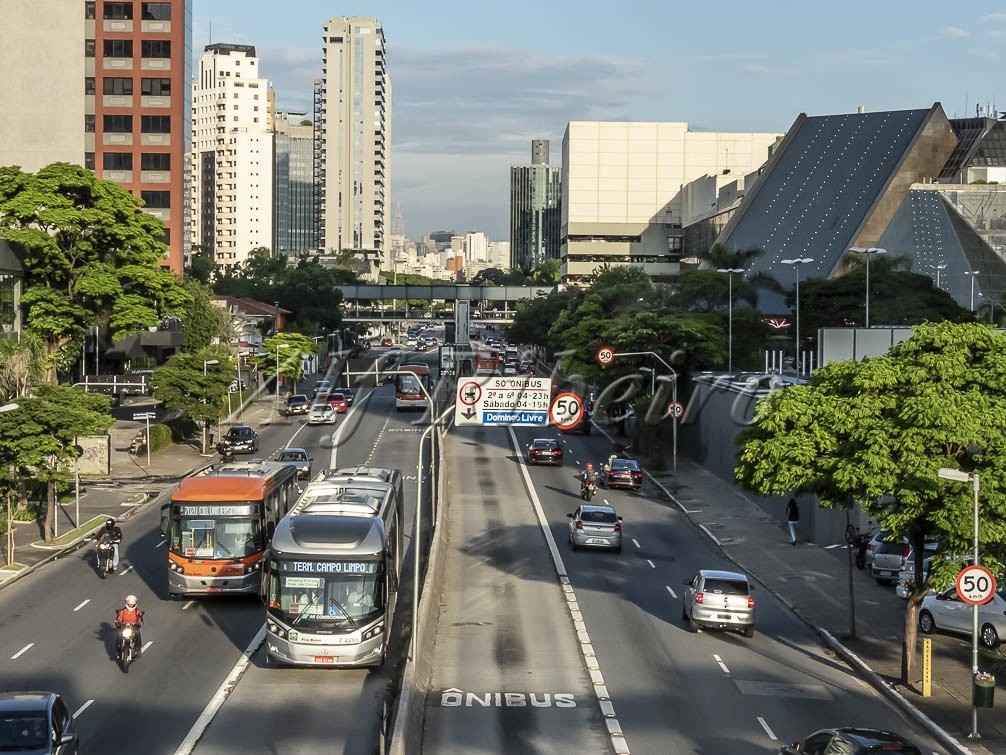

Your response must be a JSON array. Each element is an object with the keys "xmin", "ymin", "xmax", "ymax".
[
  {"xmin": 0, "ymin": 680, "xmax": 79, "ymax": 755},
  {"xmin": 526, "ymin": 438, "xmax": 566, "ymax": 466},
  {"xmin": 483, "ymin": 324, "xmax": 535, "ymax": 378},
  {"xmin": 366, "ymin": 322, "xmax": 443, "ymax": 382},
  {"xmin": 599, "ymin": 458, "xmax": 642, "ymax": 491},
  {"xmin": 866, "ymin": 526, "xmax": 1005, "ymax": 649},
  {"xmin": 777, "ymin": 727, "xmax": 923, "ymax": 755},
  {"xmin": 568, "ymin": 504, "xmax": 623, "ymax": 552},
  {"xmin": 222, "ymin": 381, "xmax": 354, "ymax": 480}
]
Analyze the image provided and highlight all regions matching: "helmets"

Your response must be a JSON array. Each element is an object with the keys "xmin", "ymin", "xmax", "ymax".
[
  {"xmin": 585, "ymin": 463, "xmax": 592, "ymax": 470},
  {"xmin": 106, "ymin": 518, "xmax": 115, "ymax": 529},
  {"xmin": 124, "ymin": 594, "xmax": 138, "ymax": 612}
]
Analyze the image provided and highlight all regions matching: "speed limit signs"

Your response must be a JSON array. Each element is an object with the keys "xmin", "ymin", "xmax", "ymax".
[
  {"xmin": 550, "ymin": 393, "xmax": 584, "ymax": 429},
  {"xmin": 670, "ymin": 403, "xmax": 683, "ymax": 417},
  {"xmin": 599, "ymin": 348, "xmax": 613, "ymax": 365}
]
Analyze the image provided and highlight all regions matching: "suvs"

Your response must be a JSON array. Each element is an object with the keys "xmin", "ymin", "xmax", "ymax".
[{"xmin": 683, "ymin": 569, "xmax": 753, "ymax": 638}]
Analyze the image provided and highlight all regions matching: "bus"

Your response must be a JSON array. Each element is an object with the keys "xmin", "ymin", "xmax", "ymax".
[
  {"xmin": 476, "ymin": 346, "xmax": 499, "ymax": 375},
  {"xmin": 159, "ymin": 458, "xmax": 404, "ymax": 668},
  {"xmin": 389, "ymin": 362, "xmax": 433, "ymax": 411}
]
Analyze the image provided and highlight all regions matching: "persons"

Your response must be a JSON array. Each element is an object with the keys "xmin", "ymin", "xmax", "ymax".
[
  {"xmin": 295, "ymin": 453, "xmax": 306, "ymax": 461},
  {"xmin": 786, "ymin": 498, "xmax": 800, "ymax": 545},
  {"xmin": 220, "ymin": 447, "xmax": 237, "ymax": 463},
  {"xmin": 115, "ymin": 594, "xmax": 143, "ymax": 657},
  {"xmin": 234, "ymin": 533, "xmax": 256, "ymax": 549},
  {"xmin": 579, "ymin": 462, "xmax": 597, "ymax": 494},
  {"xmin": 20, "ymin": 718, "xmax": 56, "ymax": 743},
  {"xmin": 346, "ymin": 582, "xmax": 375, "ymax": 607},
  {"xmin": 93, "ymin": 518, "xmax": 123, "ymax": 570}
]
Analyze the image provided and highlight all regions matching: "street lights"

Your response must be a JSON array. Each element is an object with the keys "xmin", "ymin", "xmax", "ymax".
[
  {"xmin": 718, "ymin": 248, "xmax": 1002, "ymax": 400},
  {"xmin": 642, "ymin": 366, "xmax": 678, "ymax": 484}
]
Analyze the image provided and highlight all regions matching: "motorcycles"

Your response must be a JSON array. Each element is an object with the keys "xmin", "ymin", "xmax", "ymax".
[
  {"xmin": 88, "ymin": 531, "xmax": 146, "ymax": 672},
  {"xmin": 580, "ymin": 472, "xmax": 598, "ymax": 500}
]
